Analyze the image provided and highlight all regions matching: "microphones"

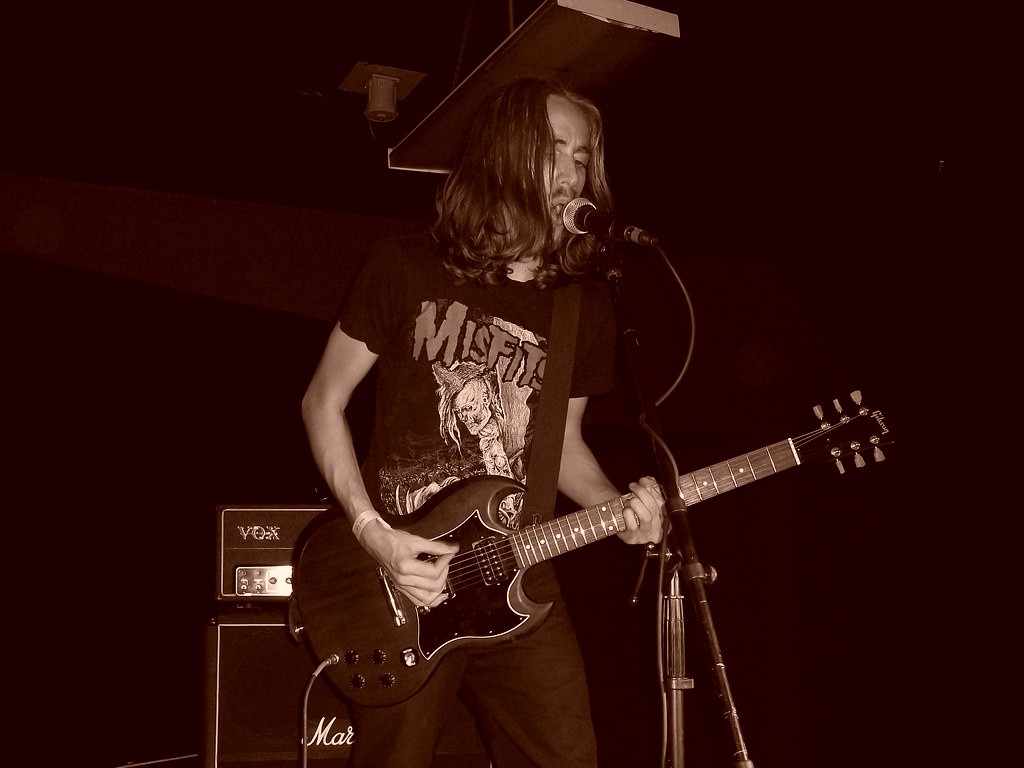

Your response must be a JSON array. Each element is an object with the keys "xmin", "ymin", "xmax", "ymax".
[{"xmin": 561, "ymin": 197, "xmax": 658, "ymax": 248}]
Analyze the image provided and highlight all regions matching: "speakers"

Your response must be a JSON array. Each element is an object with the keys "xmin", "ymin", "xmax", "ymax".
[{"xmin": 201, "ymin": 613, "xmax": 355, "ymax": 768}]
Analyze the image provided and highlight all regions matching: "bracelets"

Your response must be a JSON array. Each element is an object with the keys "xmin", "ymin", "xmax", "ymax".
[{"xmin": 352, "ymin": 508, "xmax": 381, "ymax": 544}]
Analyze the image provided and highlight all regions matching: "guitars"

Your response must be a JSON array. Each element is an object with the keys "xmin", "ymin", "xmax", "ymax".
[{"xmin": 287, "ymin": 387, "xmax": 899, "ymax": 709}]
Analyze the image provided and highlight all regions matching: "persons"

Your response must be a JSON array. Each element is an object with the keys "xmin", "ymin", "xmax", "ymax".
[{"xmin": 303, "ymin": 66, "xmax": 668, "ymax": 767}]
